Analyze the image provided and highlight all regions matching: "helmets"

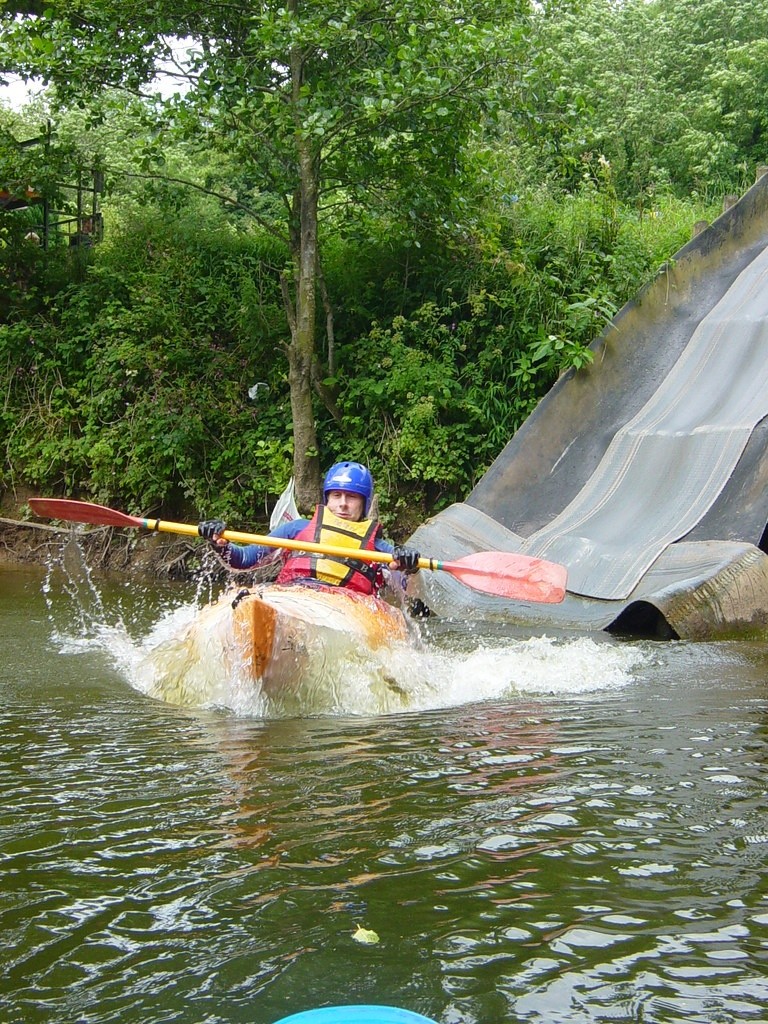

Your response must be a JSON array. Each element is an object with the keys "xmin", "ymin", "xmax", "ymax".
[{"xmin": 322, "ymin": 462, "xmax": 373, "ymax": 517}]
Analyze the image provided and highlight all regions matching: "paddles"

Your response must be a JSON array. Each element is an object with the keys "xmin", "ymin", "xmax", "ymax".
[{"xmin": 26, "ymin": 500, "xmax": 571, "ymax": 606}]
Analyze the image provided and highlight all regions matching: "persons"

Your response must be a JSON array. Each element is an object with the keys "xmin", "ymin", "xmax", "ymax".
[{"xmin": 198, "ymin": 462, "xmax": 420, "ymax": 598}]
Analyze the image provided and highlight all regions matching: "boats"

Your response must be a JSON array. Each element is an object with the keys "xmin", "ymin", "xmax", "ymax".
[{"xmin": 166, "ymin": 582, "xmax": 409, "ymax": 703}]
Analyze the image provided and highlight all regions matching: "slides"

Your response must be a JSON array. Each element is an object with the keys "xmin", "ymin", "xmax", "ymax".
[{"xmin": 401, "ymin": 175, "xmax": 768, "ymax": 633}]
